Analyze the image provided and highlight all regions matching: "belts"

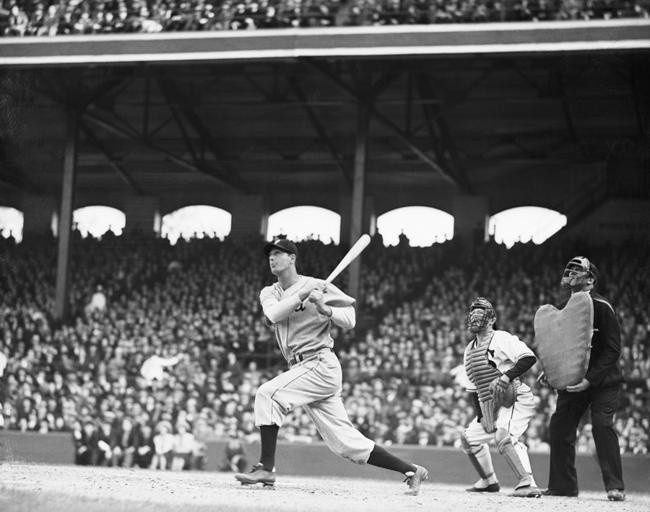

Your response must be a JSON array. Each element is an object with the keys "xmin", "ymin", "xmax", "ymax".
[{"xmin": 289, "ymin": 351, "xmax": 314, "ymax": 367}]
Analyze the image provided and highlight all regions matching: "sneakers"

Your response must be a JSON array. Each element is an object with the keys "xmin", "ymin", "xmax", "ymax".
[
  {"xmin": 403, "ymin": 464, "xmax": 428, "ymax": 494},
  {"xmin": 236, "ymin": 463, "xmax": 275, "ymax": 486},
  {"xmin": 466, "ymin": 478, "xmax": 499, "ymax": 492},
  {"xmin": 515, "ymin": 477, "xmax": 578, "ymax": 497},
  {"xmin": 608, "ymin": 488, "xmax": 625, "ymax": 500}
]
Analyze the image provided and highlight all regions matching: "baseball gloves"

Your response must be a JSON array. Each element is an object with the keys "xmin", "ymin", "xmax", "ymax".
[{"xmin": 488, "ymin": 376, "xmax": 517, "ymax": 407}]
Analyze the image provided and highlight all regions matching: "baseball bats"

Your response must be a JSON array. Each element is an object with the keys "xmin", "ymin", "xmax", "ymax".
[{"xmin": 302, "ymin": 234, "xmax": 371, "ymax": 303}]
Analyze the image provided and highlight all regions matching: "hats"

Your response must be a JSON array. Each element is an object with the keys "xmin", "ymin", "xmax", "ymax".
[{"xmin": 264, "ymin": 238, "xmax": 297, "ymax": 256}]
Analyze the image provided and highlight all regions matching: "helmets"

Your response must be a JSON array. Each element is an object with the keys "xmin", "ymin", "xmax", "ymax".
[
  {"xmin": 465, "ymin": 297, "xmax": 496, "ymax": 332},
  {"xmin": 561, "ymin": 256, "xmax": 600, "ymax": 296}
]
